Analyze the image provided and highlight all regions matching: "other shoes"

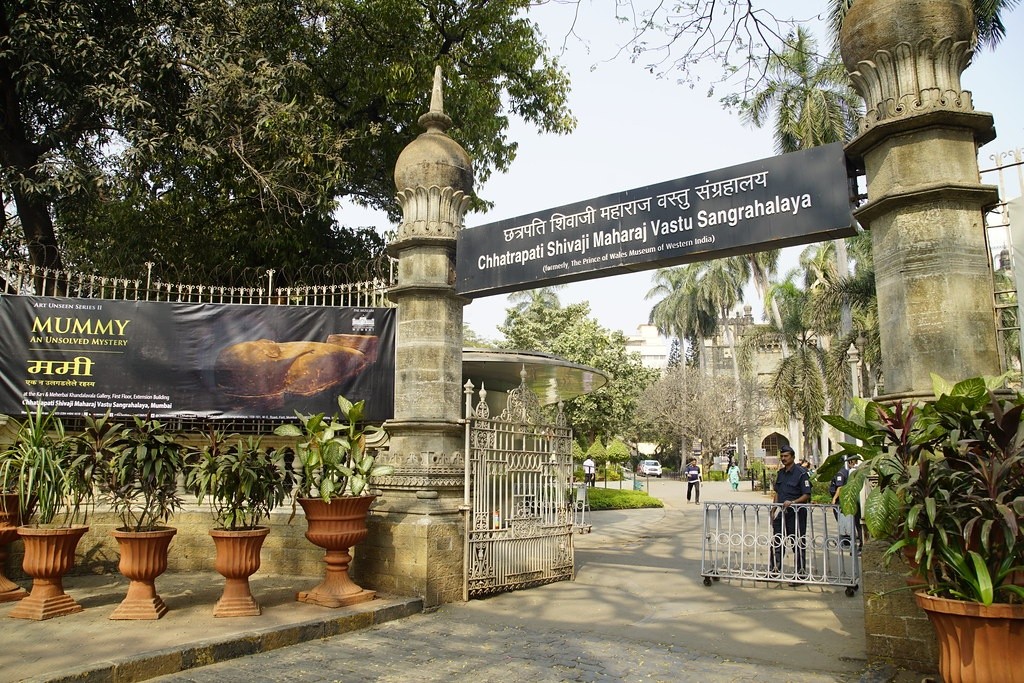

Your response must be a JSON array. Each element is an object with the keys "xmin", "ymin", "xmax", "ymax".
[
  {"xmin": 686, "ymin": 499, "xmax": 690, "ymax": 504},
  {"xmin": 758, "ymin": 572, "xmax": 781, "ymax": 583},
  {"xmin": 788, "ymin": 574, "xmax": 804, "ymax": 586},
  {"xmin": 694, "ymin": 500, "xmax": 700, "ymax": 505}
]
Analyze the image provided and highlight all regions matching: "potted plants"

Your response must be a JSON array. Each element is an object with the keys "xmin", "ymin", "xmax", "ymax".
[
  {"xmin": 98, "ymin": 414, "xmax": 182, "ymax": 619},
  {"xmin": 1, "ymin": 410, "xmax": 98, "ymax": 620},
  {"xmin": 187, "ymin": 426, "xmax": 286, "ymax": 619},
  {"xmin": 277, "ymin": 389, "xmax": 392, "ymax": 612},
  {"xmin": 0, "ymin": 460, "xmax": 41, "ymax": 600},
  {"xmin": 794, "ymin": 369, "xmax": 1024, "ymax": 683}
]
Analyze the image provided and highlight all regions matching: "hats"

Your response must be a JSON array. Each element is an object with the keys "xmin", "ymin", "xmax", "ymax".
[{"xmin": 780, "ymin": 445, "xmax": 793, "ymax": 452}]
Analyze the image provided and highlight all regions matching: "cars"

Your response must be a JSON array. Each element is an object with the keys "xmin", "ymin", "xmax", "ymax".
[
  {"xmin": 641, "ymin": 459, "xmax": 662, "ymax": 476},
  {"xmin": 636, "ymin": 460, "xmax": 649, "ymax": 477}
]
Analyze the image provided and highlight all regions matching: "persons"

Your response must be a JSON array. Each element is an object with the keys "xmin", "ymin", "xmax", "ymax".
[
  {"xmin": 684, "ymin": 457, "xmax": 703, "ymax": 505},
  {"xmin": 727, "ymin": 460, "xmax": 741, "ymax": 492},
  {"xmin": 583, "ymin": 455, "xmax": 595, "ymax": 487},
  {"xmin": 848, "ymin": 460, "xmax": 869, "ymax": 545},
  {"xmin": 830, "ymin": 457, "xmax": 851, "ymax": 546},
  {"xmin": 756, "ymin": 445, "xmax": 813, "ymax": 587}
]
